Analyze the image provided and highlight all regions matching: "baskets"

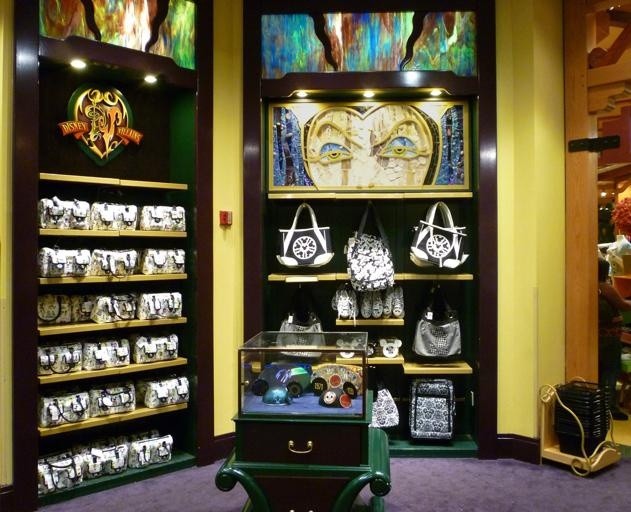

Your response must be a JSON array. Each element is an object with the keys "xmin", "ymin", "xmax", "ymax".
[{"xmin": 555, "ymin": 380, "xmax": 610, "ymax": 459}]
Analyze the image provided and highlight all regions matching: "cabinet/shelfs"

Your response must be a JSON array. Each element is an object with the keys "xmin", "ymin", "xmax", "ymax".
[
  {"xmin": 252, "ymin": 273, "xmax": 480, "ymax": 459},
  {"xmin": 30, "ymin": 171, "xmax": 190, "ymax": 509}
]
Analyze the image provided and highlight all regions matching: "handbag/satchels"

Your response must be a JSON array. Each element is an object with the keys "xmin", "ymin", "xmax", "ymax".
[
  {"xmin": 413, "ymin": 320, "xmax": 463, "ymax": 358},
  {"xmin": 39, "ymin": 245, "xmax": 186, "ymax": 279},
  {"xmin": 138, "ymin": 206, "xmax": 187, "ymax": 233},
  {"xmin": 276, "ymin": 226, "xmax": 334, "ymax": 269},
  {"xmin": 368, "ymin": 390, "xmax": 400, "ymax": 427},
  {"xmin": 38, "ymin": 197, "xmax": 91, "ymax": 231},
  {"xmin": 91, "ymin": 202, "xmax": 139, "ymax": 232},
  {"xmin": 407, "ymin": 220, "xmax": 469, "ymax": 269},
  {"xmin": 36, "ymin": 293, "xmax": 190, "ymax": 428},
  {"xmin": 276, "ymin": 321, "xmax": 326, "ymax": 359},
  {"xmin": 36, "ymin": 429, "xmax": 174, "ymax": 498},
  {"xmin": 345, "ymin": 233, "xmax": 394, "ymax": 292}
]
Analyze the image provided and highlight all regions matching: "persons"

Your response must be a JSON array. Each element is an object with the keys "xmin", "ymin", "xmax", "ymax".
[{"xmin": 598, "ymin": 257, "xmax": 631, "ymax": 420}]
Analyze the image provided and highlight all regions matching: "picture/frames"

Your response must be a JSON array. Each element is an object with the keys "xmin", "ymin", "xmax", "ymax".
[{"xmin": 269, "ymin": 100, "xmax": 471, "ymax": 194}]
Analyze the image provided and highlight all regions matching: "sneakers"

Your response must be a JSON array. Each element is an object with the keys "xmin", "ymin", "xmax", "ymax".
[
  {"xmin": 611, "ymin": 407, "xmax": 628, "ymax": 420},
  {"xmin": 332, "ymin": 285, "xmax": 405, "ymax": 319}
]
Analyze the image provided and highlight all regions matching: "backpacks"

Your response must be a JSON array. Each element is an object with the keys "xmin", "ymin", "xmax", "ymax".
[{"xmin": 408, "ymin": 378, "xmax": 458, "ymax": 440}]
